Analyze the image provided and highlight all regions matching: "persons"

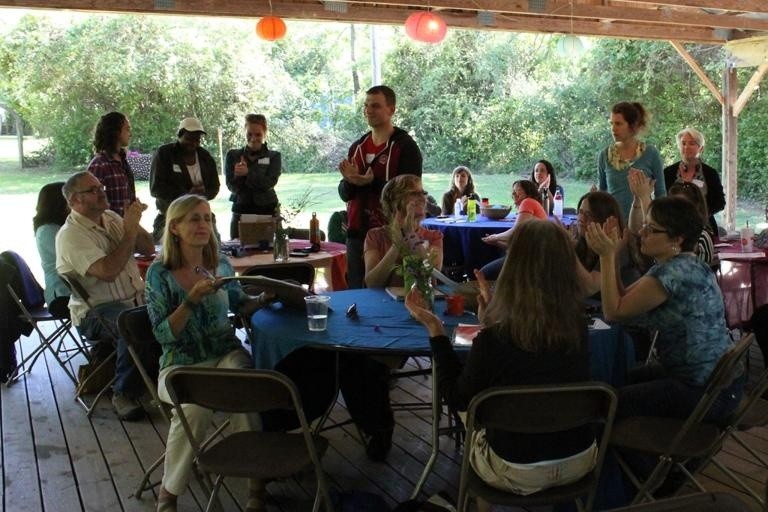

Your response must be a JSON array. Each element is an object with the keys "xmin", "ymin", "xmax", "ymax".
[
  {"xmin": 480, "ymin": 159, "xmax": 564, "ymax": 282},
  {"xmin": 225, "ymin": 114, "xmax": 282, "ymax": 242},
  {"xmin": 582, "ymin": 196, "xmax": 734, "ymax": 503},
  {"xmin": 444, "ymin": 165, "xmax": 481, "ymax": 216},
  {"xmin": 86, "ymin": 111, "xmax": 138, "ymax": 219},
  {"xmin": 577, "ymin": 102, "xmax": 666, "ymax": 235},
  {"xmin": 337, "ymin": 85, "xmax": 422, "ymax": 290},
  {"xmin": 142, "ymin": 193, "xmax": 284, "ymax": 510},
  {"xmin": 148, "ymin": 117, "xmax": 221, "ymax": 249},
  {"xmin": 32, "ymin": 171, "xmax": 154, "ymax": 422},
  {"xmin": 362, "ymin": 174, "xmax": 443, "ymax": 288},
  {"xmin": 663, "ymin": 127, "xmax": 727, "ymax": 244},
  {"xmin": 400, "ymin": 219, "xmax": 601, "ymax": 512}
]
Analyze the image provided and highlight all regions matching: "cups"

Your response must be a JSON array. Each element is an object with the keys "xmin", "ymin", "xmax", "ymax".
[
  {"xmin": 739, "ymin": 228, "xmax": 755, "ymax": 252},
  {"xmin": 304, "ymin": 294, "xmax": 331, "ymax": 333},
  {"xmin": 445, "ymin": 295, "xmax": 465, "ymax": 317}
]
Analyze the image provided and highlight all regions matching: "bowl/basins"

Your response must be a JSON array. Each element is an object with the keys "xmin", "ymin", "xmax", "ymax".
[
  {"xmin": 479, "ymin": 205, "xmax": 512, "ymax": 220},
  {"xmin": 455, "ymin": 281, "xmax": 497, "ymax": 314}
]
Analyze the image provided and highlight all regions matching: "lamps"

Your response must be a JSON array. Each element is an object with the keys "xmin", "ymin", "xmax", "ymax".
[{"xmin": 256, "ymin": 1, "xmax": 285, "ymax": 41}]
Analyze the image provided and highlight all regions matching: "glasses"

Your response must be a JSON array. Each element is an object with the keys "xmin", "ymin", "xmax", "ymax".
[
  {"xmin": 641, "ymin": 224, "xmax": 666, "ymax": 235},
  {"xmin": 77, "ymin": 185, "xmax": 107, "ymax": 195},
  {"xmin": 404, "ymin": 190, "xmax": 429, "ymax": 197}
]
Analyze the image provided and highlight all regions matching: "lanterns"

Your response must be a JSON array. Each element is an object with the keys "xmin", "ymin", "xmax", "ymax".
[{"xmin": 255, "ymin": 16, "xmax": 288, "ymax": 41}]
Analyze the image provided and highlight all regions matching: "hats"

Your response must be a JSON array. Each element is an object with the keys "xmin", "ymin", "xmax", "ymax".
[{"xmin": 180, "ymin": 118, "xmax": 207, "ymax": 135}]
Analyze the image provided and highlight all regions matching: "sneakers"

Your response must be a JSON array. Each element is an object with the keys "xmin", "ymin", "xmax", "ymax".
[
  {"xmin": 111, "ymin": 394, "xmax": 146, "ymax": 421},
  {"xmin": 366, "ymin": 435, "xmax": 392, "ymax": 462},
  {"xmin": 78, "ymin": 365, "xmax": 88, "ymax": 392}
]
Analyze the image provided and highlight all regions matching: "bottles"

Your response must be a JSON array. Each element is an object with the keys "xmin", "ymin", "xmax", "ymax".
[
  {"xmin": 541, "ymin": 188, "xmax": 563, "ymax": 218},
  {"xmin": 307, "ymin": 211, "xmax": 321, "ymax": 246},
  {"xmin": 453, "ymin": 199, "xmax": 463, "ymax": 218},
  {"xmin": 466, "ymin": 194, "xmax": 476, "ymax": 223}
]
